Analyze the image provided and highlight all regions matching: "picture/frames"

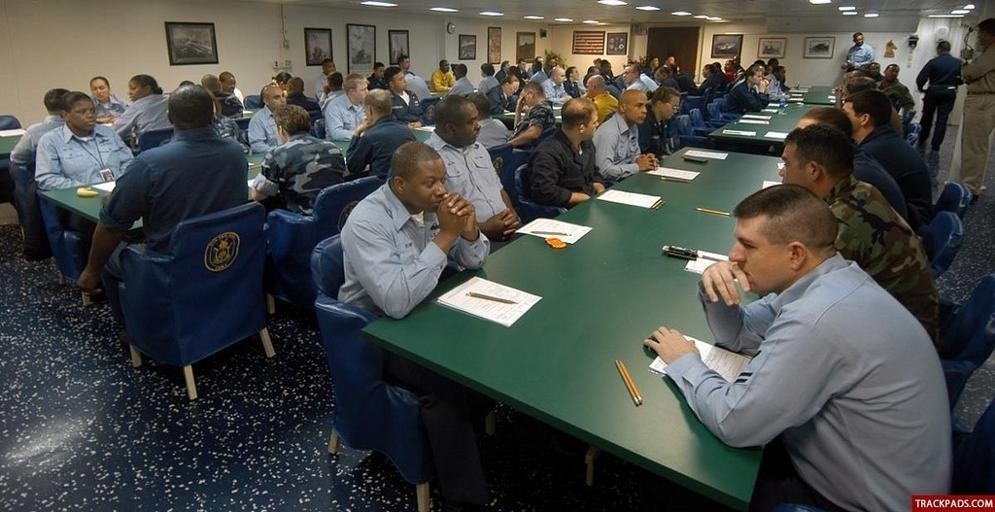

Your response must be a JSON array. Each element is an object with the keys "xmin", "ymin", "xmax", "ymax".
[
  {"xmin": 304, "ymin": 27, "xmax": 333, "ymax": 67},
  {"xmin": 803, "ymin": 37, "xmax": 835, "ymax": 59},
  {"xmin": 607, "ymin": 32, "xmax": 627, "ymax": 55},
  {"xmin": 515, "ymin": 31, "xmax": 535, "ymax": 63},
  {"xmin": 388, "ymin": 28, "xmax": 410, "ymax": 66},
  {"xmin": 711, "ymin": 34, "xmax": 743, "ymax": 58},
  {"xmin": 459, "ymin": 34, "xmax": 477, "ymax": 61},
  {"xmin": 487, "ymin": 26, "xmax": 501, "ymax": 65},
  {"xmin": 757, "ymin": 38, "xmax": 787, "ymax": 59},
  {"xmin": 164, "ymin": 20, "xmax": 219, "ymax": 65},
  {"xmin": 346, "ymin": 23, "xmax": 377, "ymax": 76}
]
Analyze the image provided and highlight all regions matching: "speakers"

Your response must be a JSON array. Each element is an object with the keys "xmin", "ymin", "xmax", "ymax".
[{"xmin": 540, "ymin": 28, "xmax": 547, "ymax": 37}]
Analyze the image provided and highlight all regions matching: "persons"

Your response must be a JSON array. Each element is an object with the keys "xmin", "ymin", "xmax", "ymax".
[
  {"xmin": 946, "ymin": 18, "xmax": 995, "ymax": 205},
  {"xmin": 508, "ymin": 82, "xmax": 556, "ymax": 144},
  {"xmin": 645, "ymin": 185, "xmax": 952, "ymax": 511},
  {"xmin": 286, "ymin": 78, "xmax": 320, "ymax": 111},
  {"xmin": 544, "ymin": 57, "xmax": 556, "ymax": 73},
  {"xmin": 507, "ymin": 66, "xmax": 526, "ymax": 86},
  {"xmin": 867, "ymin": 62, "xmax": 881, "ymax": 81},
  {"xmin": 877, "ymin": 63, "xmax": 915, "ymax": 116},
  {"xmin": 382, "ymin": 67, "xmax": 421, "ymax": 118},
  {"xmin": 368, "ymin": 61, "xmax": 386, "ymax": 87},
  {"xmin": 766, "ymin": 66, "xmax": 786, "ymax": 100},
  {"xmin": 841, "ymin": 91, "xmax": 933, "ymax": 220},
  {"xmin": 345, "ymin": 88, "xmax": 416, "ymax": 178},
  {"xmin": 320, "ymin": 72, "xmax": 343, "ymax": 106},
  {"xmin": 541, "ymin": 68, "xmax": 571, "ymax": 100},
  {"xmin": 430, "ymin": 60, "xmax": 455, "ymax": 92},
  {"xmin": 486, "ymin": 76, "xmax": 519, "ymax": 114},
  {"xmin": 530, "ymin": 62, "xmax": 546, "ymax": 83},
  {"xmin": 564, "ymin": 67, "xmax": 581, "ymax": 97},
  {"xmin": 592, "ymin": 90, "xmax": 659, "ymax": 181},
  {"xmin": 87, "ymin": 76, "xmax": 131, "ymax": 123},
  {"xmin": 639, "ymin": 87, "xmax": 681, "ymax": 160},
  {"xmin": 517, "ymin": 58, "xmax": 528, "ymax": 78},
  {"xmin": 798, "ymin": 107, "xmax": 907, "ymax": 224},
  {"xmin": 449, "ymin": 64, "xmax": 474, "ymax": 96},
  {"xmin": 495, "ymin": 60, "xmax": 510, "ymax": 82},
  {"xmin": 9, "ymin": 89, "xmax": 72, "ymax": 167},
  {"xmin": 252, "ymin": 103, "xmax": 345, "ymax": 216},
  {"xmin": 767, "ymin": 58, "xmax": 778, "ymax": 68},
  {"xmin": 75, "ymin": 84, "xmax": 248, "ymax": 325},
  {"xmin": 276, "ymin": 71, "xmax": 290, "ymax": 89},
  {"xmin": 398, "ymin": 54, "xmax": 415, "ymax": 76},
  {"xmin": 844, "ymin": 76, "xmax": 902, "ymax": 131},
  {"xmin": 713, "ymin": 62, "xmax": 728, "ymax": 86},
  {"xmin": 465, "ymin": 92, "xmax": 508, "ymax": 146},
  {"xmin": 477, "ymin": 63, "xmax": 499, "ymax": 93},
  {"xmin": 316, "ymin": 59, "xmax": 340, "ymax": 99},
  {"xmin": 219, "ymin": 71, "xmax": 246, "ymax": 111},
  {"xmin": 35, "ymin": 91, "xmax": 134, "ymax": 190},
  {"xmin": 916, "ymin": 41, "xmax": 965, "ymax": 151},
  {"xmin": 699, "ymin": 64, "xmax": 716, "ymax": 91},
  {"xmin": 325, "ymin": 73, "xmax": 370, "ymax": 139},
  {"xmin": 724, "ymin": 60, "xmax": 735, "ymax": 79},
  {"xmin": 842, "ymin": 31, "xmax": 875, "ymax": 70},
  {"xmin": 423, "ymin": 94, "xmax": 520, "ymax": 243},
  {"xmin": 338, "ymin": 142, "xmax": 489, "ymax": 512},
  {"xmin": 523, "ymin": 96, "xmax": 607, "ymax": 209},
  {"xmin": 201, "ymin": 73, "xmax": 243, "ymax": 114},
  {"xmin": 779, "ymin": 124, "xmax": 940, "ymax": 346},
  {"xmin": 527, "ymin": 56, "xmax": 545, "ymax": 75},
  {"xmin": 728, "ymin": 68, "xmax": 769, "ymax": 114},
  {"xmin": 732, "ymin": 64, "xmax": 765, "ymax": 90},
  {"xmin": 111, "ymin": 74, "xmax": 169, "ymax": 143},
  {"xmin": 248, "ymin": 82, "xmax": 287, "ymax": 152},
  {"xmin": 405, "ymin": 72, "xmax": 431, "ymax": 98},
  {"xmin": 583, "ymin": 54, "xmax": 696, "ymax": 96},
  {"xmin": 733, "ymin": 56, "xmax": 744, "ymax": 74}
]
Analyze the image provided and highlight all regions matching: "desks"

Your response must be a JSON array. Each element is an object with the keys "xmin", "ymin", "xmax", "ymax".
[
  {"xmin": 778, "ymin": 86, "xmax": 838, "ymax": 104},
  {"xmin": 359, "ymin": 145, "xmax": 784, "ymax": 512},
  {"xmin": 707, "ymin": 103, "xmax": 836, "ymax": 151},
  {"xmin": 0, "ymin": 107, "xmax": 267, "ymax": 159},
  {"xmin": 492, "ymin": 102, "xmax": 561, "ymax": 125},
  {"xmin": 41, "ymin": 127, "xmax": 432, "ymax": 231}
]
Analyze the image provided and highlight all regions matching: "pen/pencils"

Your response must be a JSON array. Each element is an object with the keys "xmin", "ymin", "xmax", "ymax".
[
  {"xmin": 696, "ymin": 207, "xmax": 730, "ymax": 218},
  {"xmin": 466, "ymin": 291, "xmax": 518, "ymax": 304},
  {"xmin": 651, "ymin": 199, "xmax": 666, "ymax": 210},
  {"xmin": 531, "ymin": 230, "xmax": 571, "ymax": 238},
  {"xmin": 661, "ymin": 177, "xmax": 690, "ymax": 186},
  {"xmin": 614, "ymin": 358, "xmax": 643, "ymax": 408},
  {"xmin": 698, "ymin": 253, "xmax": 725, "ymax": 263}
]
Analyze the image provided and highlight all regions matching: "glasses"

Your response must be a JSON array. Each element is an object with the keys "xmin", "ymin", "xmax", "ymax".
[{"xmin": 668, "ymin": 102, "xmax": 680, "ymax": 110}]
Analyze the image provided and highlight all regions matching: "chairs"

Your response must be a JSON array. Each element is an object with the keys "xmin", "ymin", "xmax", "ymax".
[
  {"xmin": 485, "ymin": 143, "xmax": 512, "ymax": 179},
  {"xmin": 707, "ymin": 101, "xmax": 736, "ymax": 124},
  {"xmin": 767, "ymin": 400, "xmax": 994, "ymax": 511},
  {"xmin": 121, "ymin": 200, "xmax": 276, "ymax": 401},
  {"xmin": 921, "ymin": 211, "xmax": 963, "ymax": 281},
  {"xmin": 267, "ymin": 176, "xmax": 379, "ymax": 315},
  {"xmin": 511, "ymin": 161, "xmax": 568, "ymax": 220},
  {"xmin": 676, "ymin": 114, "xmax": 712, "ymax": 148},
  {"xmin": 936, "ymin": 277, "xmax": 995, "ymax": 411},
  {"xmin": 308, "ymin": 234, "xmax": 431, "ymax": 511},
  {"xmin": 714, "ymin": 98, "xmax": 741, "ymax": 121},
  {"xmin": 690, "ymin": 107, "xmax": 725, "ymax": 133},
  {"xmin": 933, "ymin": 181, "xmax": 972, "ymax": 218}
]
